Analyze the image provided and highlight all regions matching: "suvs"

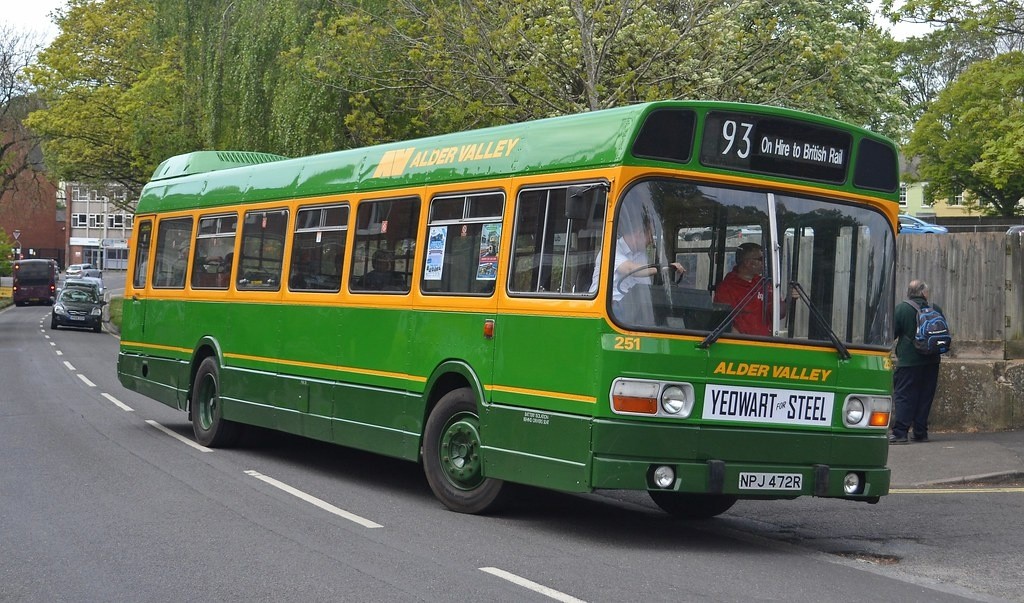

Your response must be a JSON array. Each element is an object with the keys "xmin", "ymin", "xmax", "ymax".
[{"xmin": 65, "ymin": 263, "xmax": 103, "ymax": 279}]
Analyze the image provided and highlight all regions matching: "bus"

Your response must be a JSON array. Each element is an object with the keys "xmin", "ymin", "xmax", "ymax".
[
  {"xmin": 12, "ymin": 258, "xmax": 57, "ymax": 307},
  {"xmin": 117, "ymin": 97, "xmax": 900, "ymax": 521}
]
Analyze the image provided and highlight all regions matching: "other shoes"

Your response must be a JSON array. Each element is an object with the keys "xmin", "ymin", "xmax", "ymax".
[
  {"xmin": 887, "ymin": 434, "xmax": 908, "ymax": 445},
  {"xmin": 911, "ymin": 434, "xmax": 928, "ymax": 442}
]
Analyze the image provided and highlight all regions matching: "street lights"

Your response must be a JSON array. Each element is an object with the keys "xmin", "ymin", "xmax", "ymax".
[{"xmin": 12, "ymin": 229, "xmax": 21, "ymax": 260}]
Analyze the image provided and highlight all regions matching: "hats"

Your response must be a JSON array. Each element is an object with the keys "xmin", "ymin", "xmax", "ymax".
[{"xmin": 179, "ymin": 239, "xmax": 191, "ymax": 250}]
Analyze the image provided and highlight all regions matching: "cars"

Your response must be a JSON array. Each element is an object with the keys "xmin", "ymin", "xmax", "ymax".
[
  {"xmin": 863, "ymin": 214, "xmax": 949, "ymax": 234},
  {"xmin": 651, "ymin": 222, "xmax": 814, "ymax": 254},
  {"xmin": 50, "ymin": 285, "xmax": 107, "ymax": 333},
  {"xmin": 56, "ymin": 266, "xmax": 107, "ymax": 301}
]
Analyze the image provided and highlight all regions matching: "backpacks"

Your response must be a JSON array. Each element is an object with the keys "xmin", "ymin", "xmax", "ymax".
[{"xmin": 904, "ymin": 298, "xmax": 951, "ymax": 356}]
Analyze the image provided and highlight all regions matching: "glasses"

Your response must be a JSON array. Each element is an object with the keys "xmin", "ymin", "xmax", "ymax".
[{"xmin": 746, "ymin": 256, "xmax": 764, "ymax": 262}]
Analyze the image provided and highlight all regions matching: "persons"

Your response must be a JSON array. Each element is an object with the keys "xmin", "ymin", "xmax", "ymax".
[
  {"xmin": 358, "ymin": 249, "xmax": 408, "ymax": 291},
  {"xmin": 587, "ymin": 213, "xmax": 685, "ymax": 328},
  {"xmin": 220, "ymin": 253, "xmax": 245, "ymax": 287},
  {"xmin": 888, "ymin": 278, "xmax": 947, "ymax": 443},
  {"xmin": 714, "ymin": 242, "xmax": 802, "ymax": 339},
  {"xmin": 172, "ymin": 240, "xmax": 221, "ymax": 286},
  {"xmin": 139, "ymin": 242, "xmax": 162, "ymax": 286}
]
